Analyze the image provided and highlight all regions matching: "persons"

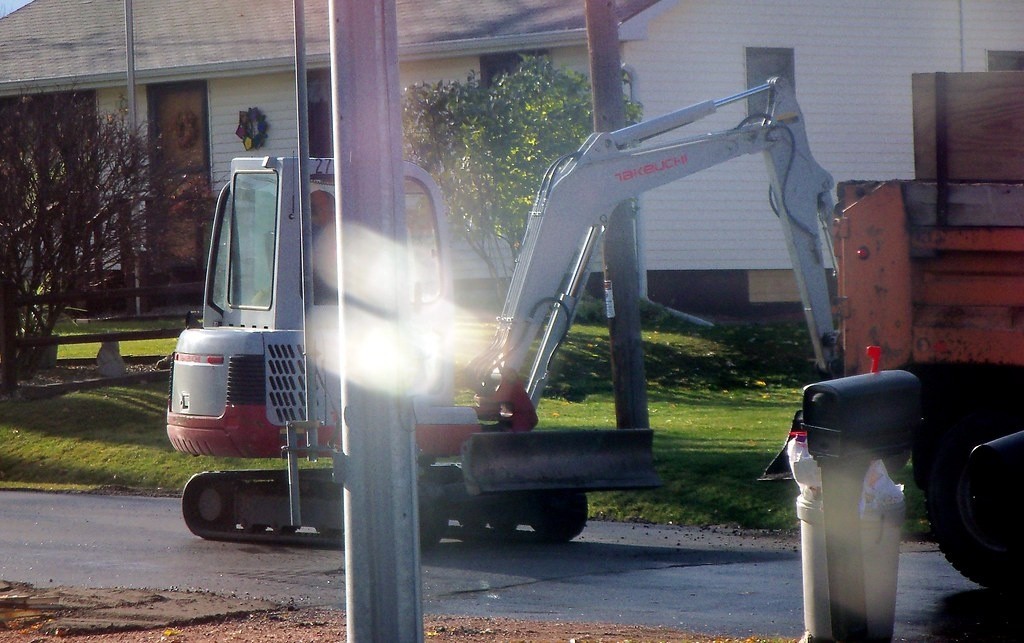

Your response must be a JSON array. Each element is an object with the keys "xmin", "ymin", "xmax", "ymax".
[{"xmin": 302, "ymin": 189, "xmax": 337, "ymax": 305}]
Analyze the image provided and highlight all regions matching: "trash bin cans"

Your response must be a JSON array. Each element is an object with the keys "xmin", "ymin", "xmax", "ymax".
[{"xmin": 796, "ymin": 498, "xmax": 904, "ymax": 643}]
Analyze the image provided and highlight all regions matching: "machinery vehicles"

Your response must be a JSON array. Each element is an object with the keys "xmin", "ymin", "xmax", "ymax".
[{"xmin": 167, "ymin": 74, "xmax": 840, "ymax": 549}]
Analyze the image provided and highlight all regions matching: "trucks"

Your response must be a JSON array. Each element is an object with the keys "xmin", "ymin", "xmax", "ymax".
[{"xmin": 823, "ymin": 179, "xmax": 1023, "ymax": 595}]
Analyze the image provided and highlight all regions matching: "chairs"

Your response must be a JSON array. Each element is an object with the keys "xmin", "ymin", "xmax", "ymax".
[{"xmin": 264, "ymin": 230, "xmax": 336, "ymax": 303}]
